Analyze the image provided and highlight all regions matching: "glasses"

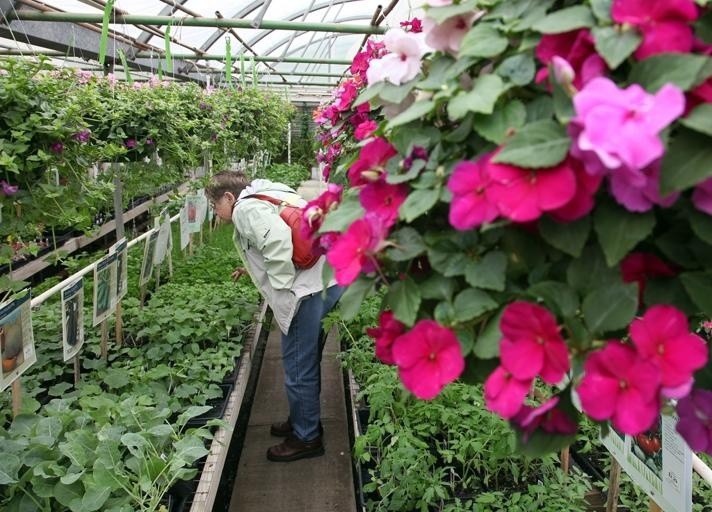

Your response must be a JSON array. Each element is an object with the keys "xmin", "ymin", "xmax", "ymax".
[{"xmin": 210, "ymin": 200, "xmax": 218, "ymax": 209}]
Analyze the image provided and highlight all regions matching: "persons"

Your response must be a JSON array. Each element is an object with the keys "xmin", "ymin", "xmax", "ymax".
[{"xmin": 205, "ymin": 169, "xmax": 351, "ymax": 462}]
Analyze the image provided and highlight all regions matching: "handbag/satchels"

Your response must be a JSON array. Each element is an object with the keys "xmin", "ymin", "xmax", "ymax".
[{"xmin": 231, "ymin": 194, "xmax": 321, "ymax": 269}]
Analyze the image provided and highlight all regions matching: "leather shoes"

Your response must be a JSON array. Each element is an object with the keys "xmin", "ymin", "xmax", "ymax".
[{"xmin": 266, "ymin": 420, "xmax": 325, "ymax": 462}]
